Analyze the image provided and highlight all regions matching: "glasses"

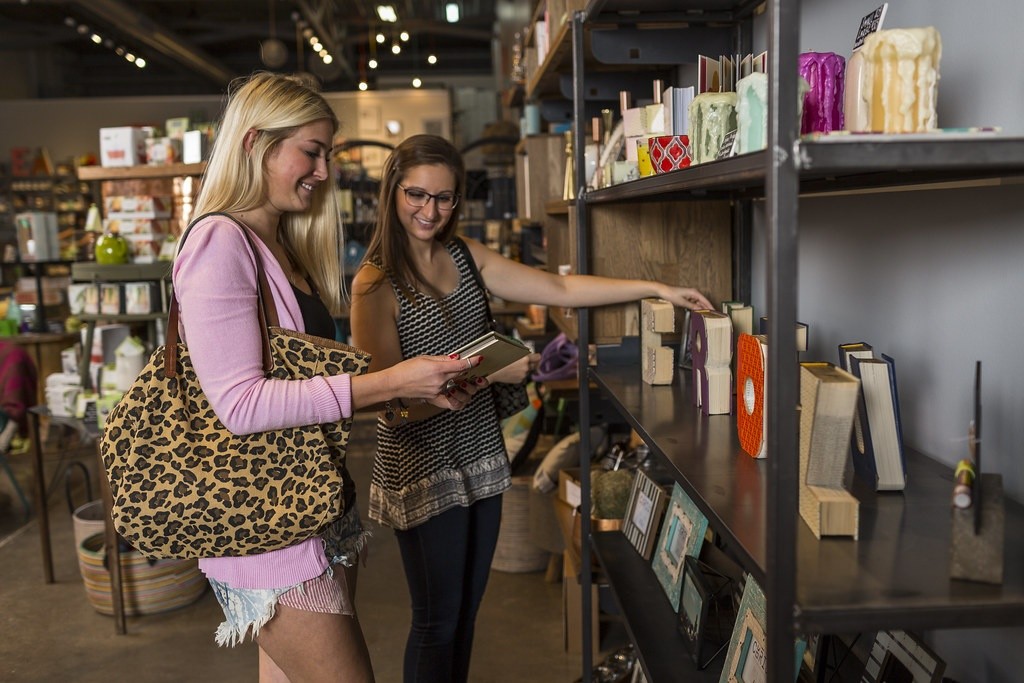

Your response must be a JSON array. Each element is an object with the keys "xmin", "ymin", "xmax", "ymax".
[{"xmin": 397, "ymin": 182, "xmax": 461, "ymax": 211}]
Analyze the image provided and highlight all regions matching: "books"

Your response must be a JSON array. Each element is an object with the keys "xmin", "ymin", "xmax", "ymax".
[
  {"xmin": 638, "ymin": 296, "xmax": 906, "ymax": 539},
  {"xmin": 450, "ymin": 331, "xmax": 531, "ymax": 383},
  {"xmin": 590, "ymin": 50, "xmax": 768, "ymax": 188}
]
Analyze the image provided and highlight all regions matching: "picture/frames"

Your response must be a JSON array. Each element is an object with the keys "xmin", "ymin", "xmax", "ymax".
[
  {"xmin": 718, "ymin": 574, "xmax": 806, "ymax": 683},
  {"xmin": 651, "ymin": 479, "xmax": 709, "ymax": 613},
  {"xmin": 677, "ymin": 554, "xmax": 713, "ymax": 671},
  {"xmin": 860, "ymin": 628, "xmax": 947, "ymax": 683},
  {"xmin": 621, "ymin": 467, "xmax": 667, "ymax": 560}
]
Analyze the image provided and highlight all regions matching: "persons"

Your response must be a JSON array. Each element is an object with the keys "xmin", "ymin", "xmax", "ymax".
[
  {"xmin": 0, "ymin": 338, "xmax": 36, "ymax": 454},
  {"xmin": 352, "ymin": 134, "xmax": 714, "ymax": 683},
  {"xmin": 172, "ymin": 72, "xmax": 489, "ymax": 683}
]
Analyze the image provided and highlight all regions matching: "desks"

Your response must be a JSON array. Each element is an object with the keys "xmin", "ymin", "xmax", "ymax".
[
  {"xmin": 28, "ymin": 405, "xmax": 128, "ymax": 635},
  {"xmin": 0, "ymin": 259, "xmax": 74, "ymax": 332}
]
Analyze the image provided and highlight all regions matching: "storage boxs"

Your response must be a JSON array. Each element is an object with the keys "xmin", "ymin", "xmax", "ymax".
[
  {"xmin": 15, "ymin": 115, "xmax": 220, "ymax": 430},
  {"xmin": 558, "ymin": 465, "xmax": 604, "ymax": 507}
]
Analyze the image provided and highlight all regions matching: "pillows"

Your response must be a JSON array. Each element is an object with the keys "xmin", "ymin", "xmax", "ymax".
[
  {"xmin": 532, "ymin": 422, "xmax": 606, "ymax": 495},
  {"xmin": 501, "ymin": 398, "xmax": 546, "ymax": 478}
]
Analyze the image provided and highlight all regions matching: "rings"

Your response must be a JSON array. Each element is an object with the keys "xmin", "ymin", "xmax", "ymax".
[
  {"xmin": 467, "ymin": 358, "xmax": 472, "ymax": 368},
  {"xmin": 447, "ymin": 379, "xmax": 454, "ymax": 388}
]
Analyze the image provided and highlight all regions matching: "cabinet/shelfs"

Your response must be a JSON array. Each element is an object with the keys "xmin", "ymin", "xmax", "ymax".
[
  {"xmin": 336, "ymin": 168, "xmax": 487, "ymax": 336},
  {"xmin": 524, "ymin": 0, "xmax": 753, "ymax": 683},
  {"xmin": 571, "ymin": 0, "xmax": 1024, "ymax": 683},
  {"xmin": 499, "ymin": 83, "xmax": 557, "ymax": 342}
]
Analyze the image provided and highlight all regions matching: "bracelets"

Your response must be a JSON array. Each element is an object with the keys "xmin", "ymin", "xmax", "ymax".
[
  {"xmin": 384, "ymin": 401, "xmax": 394, "ymax": 421},
  {"xmin": 399, "ymin": 399, "xmax": 409, "ymax": 419}
]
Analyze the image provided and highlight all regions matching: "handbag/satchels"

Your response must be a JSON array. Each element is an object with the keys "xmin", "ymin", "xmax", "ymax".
[
  {"xmin": 101, "ymin": 214, "xmax": 371, "ymax": 561},
  {"xmin": 452, "ymin": 233, "xmax": 531, "ymax": 421}
]
